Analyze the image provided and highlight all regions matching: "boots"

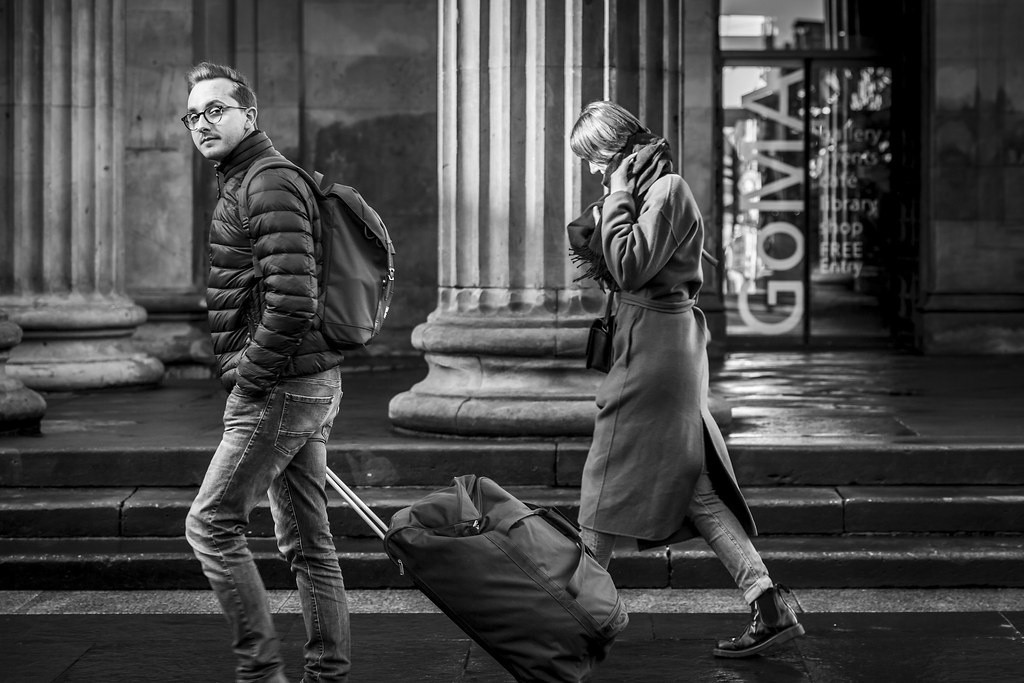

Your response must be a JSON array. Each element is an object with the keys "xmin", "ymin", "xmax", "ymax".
[{"xmin": 712, "ymin": 582, "xmax": 806, "ymax": 657}]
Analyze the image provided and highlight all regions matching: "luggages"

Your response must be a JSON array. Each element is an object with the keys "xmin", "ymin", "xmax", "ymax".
[{"xmin": 325, "ymin": 466, "xmax": 629, "ymax": 683}]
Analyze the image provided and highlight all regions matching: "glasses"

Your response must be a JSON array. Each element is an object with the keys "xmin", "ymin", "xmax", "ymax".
[{"xmin": 181, "ymin": 105, "xmax": 248, "ymax": 132}]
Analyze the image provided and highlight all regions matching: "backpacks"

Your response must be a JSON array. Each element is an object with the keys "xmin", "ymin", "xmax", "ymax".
[{"xmin": 239, "ymin": 156, "xmax": 395, "ymax": 349}]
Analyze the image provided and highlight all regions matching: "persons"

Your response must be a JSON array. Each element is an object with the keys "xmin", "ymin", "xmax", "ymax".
[
  {"xmin": 566, "ymin": 100, "xmax": 802, "ymax": 657},
  {"xmin": 179, "ymin": 62, "xmax": 354, "ymax": 683}
]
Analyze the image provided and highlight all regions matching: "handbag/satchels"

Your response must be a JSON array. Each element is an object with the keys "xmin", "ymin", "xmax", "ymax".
[{"xmin": 584, "ymin": 316, "xmax": 617, "ymax": 373}]
